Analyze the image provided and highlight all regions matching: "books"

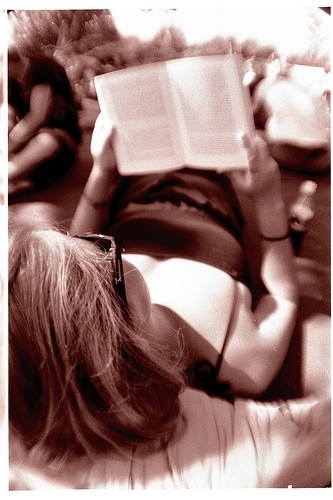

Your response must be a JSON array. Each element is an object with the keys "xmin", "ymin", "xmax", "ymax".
[{"xmin": 92, "ymin": 52, "xmax": 257, "ymax": 180}]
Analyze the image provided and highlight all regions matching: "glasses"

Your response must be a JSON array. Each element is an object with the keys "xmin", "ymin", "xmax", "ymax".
[{"xmin": 73, "ymin": 231, "xmax": 130, "ymax": 323}]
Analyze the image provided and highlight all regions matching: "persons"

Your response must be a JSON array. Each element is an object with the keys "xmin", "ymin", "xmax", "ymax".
[
  {"xmin": 10, "ymin": 105, "xmax": 300, "ymax": 469},
  {"xmin": 7, "ymin": 45, "xmax": 90, "ymax": 196}
]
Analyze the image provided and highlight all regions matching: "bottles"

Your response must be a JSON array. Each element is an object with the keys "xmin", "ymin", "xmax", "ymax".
[{"xmin": 285, "ymin": 181, "xmax": 317, "ymax": 252}]
